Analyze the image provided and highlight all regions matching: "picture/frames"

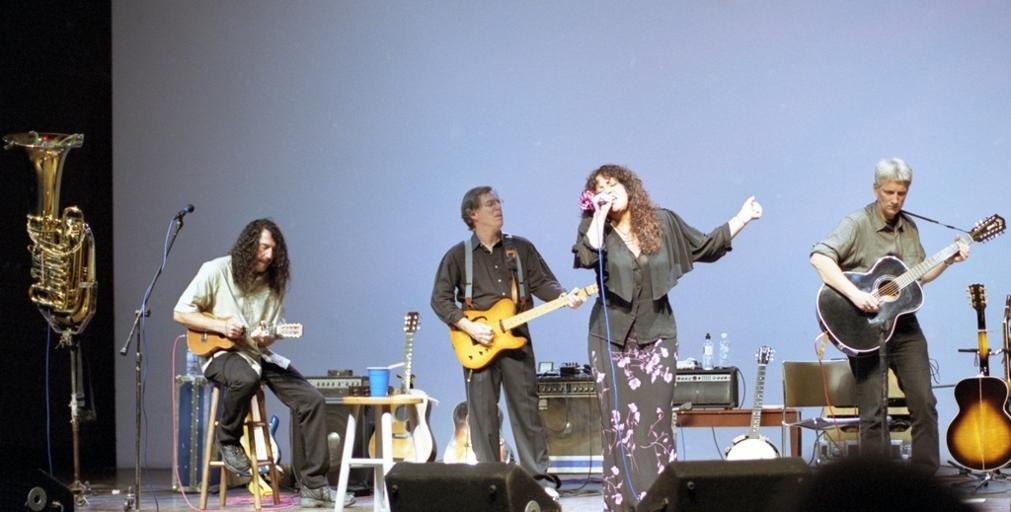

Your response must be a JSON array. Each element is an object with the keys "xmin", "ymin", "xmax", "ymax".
[{"xmin": 670, "ymin": 406, "xmax": 803, "ymax": 459}]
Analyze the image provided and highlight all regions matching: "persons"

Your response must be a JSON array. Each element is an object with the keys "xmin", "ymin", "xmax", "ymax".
[
  {"xmin": 572, "ymin": 163, "xmax": 762, "ymax": 512},
  {"xmin": 808, "ymin": 158, "xmax": 971, "ymax": 478},
  {"xmin": 443, "ymin": 401, "xmax": 514, "ymax": 465},
  {"xmin": 430, "ymin": 186, "xmax": 585, "ymax": 502},
  {"xmin": 172, "ymin": 218, "xmax": 357, "ymax": 509}
]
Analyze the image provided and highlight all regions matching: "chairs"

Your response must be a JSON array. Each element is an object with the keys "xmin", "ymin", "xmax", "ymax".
[
  {"xmin": 544, "ymin": 486, "xmax": 561, "ymax": 501},
  {"xmin": 301, "ymin": 484, "xmax": 357, "ymax": 508},
  {"xmin": 216, "ymin": 439, "xmax": 253, "ymax": 475}
]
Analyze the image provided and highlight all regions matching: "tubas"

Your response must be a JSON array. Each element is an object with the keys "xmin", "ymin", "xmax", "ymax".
[{"xmin": 5, "ymin": 131, "xmax": 96, "ymax": 335}]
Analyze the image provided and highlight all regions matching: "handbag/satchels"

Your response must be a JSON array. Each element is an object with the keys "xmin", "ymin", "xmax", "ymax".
[
  {"xmin": 734, "ymin": 215, "xmax": 746, "ymax": 226},
  {"xmin": 943, "ymin": 260, "xmax": 953, "ymax": 266}
]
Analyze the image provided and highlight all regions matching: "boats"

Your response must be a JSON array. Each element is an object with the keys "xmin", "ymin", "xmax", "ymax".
[{"xmin": 290, "ymin": 369, "xmax": 378, "ymax": 492}]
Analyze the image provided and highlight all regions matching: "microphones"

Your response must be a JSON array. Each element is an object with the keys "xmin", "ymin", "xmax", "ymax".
[
  {"xmin": 198, "ymin": 377, "xmax": 282, "ymax": 512},
  {"xmin": 331, "ymin": 393, "xmax": 427, "ymax": 512}
]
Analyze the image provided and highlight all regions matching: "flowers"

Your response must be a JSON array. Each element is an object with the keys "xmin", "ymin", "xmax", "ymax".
[
  {"xmin": 171, "ymin": 202, "xmax": 194, "ymax": 221},
  {"xmin": 598, "ymin": 192, "xmax": 615, "ymax": 206}
]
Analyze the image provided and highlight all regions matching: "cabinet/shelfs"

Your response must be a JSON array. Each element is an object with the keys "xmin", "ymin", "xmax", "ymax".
[
  {"xmin": 122, "ymin": 487, "xmax": 137, "ymax": 512},
  {"xmin": 702, "ymin": 332, "xmax": 715, "ymax": 371},
  {"xmin": 186, "ymin": 347, "xmax": 198, "ymax": 375},
  {"xmin": 719, "ymin": 332, "xmax": 734, "ymax": 369}
]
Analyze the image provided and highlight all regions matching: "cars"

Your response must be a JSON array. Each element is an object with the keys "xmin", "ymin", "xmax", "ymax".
[{"xmin": 367, "ymin": 366, "xmax": 390, "ymax": 397}]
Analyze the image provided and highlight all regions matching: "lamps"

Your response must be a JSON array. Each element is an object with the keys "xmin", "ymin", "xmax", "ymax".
[{"xmin": 781, "ymin": 358, "xmax": 894, "ymax": 464}]
